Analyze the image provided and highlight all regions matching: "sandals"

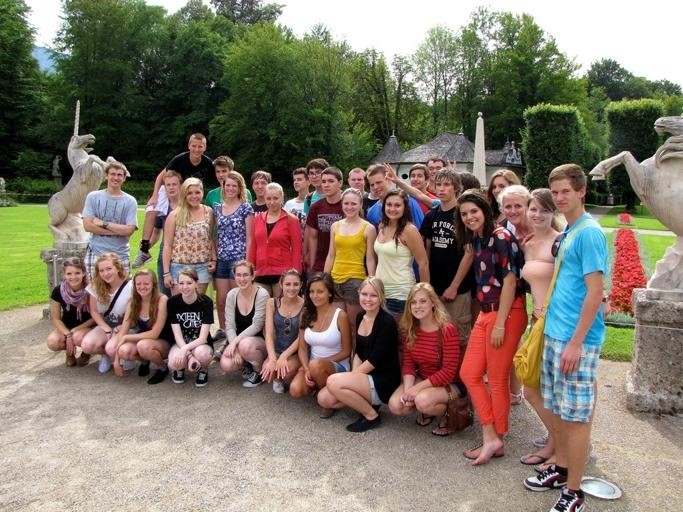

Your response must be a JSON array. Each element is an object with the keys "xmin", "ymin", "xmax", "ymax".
[{"xmin": 464, "ymin": 440, "xmax": 505, "ymax": 459}]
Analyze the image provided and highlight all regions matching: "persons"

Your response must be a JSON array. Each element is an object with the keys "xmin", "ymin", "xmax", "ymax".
[
  {"xmin": 167, "ymin": 268, "xmax": 214, "ymax": 387},
  {"xmin": 48, "ymin": 257, "xmax": 98, "ymax": 367},
  {"xmin": 259, "ymin": 269, "xmax": 305, "ymax": 393},
  {"xmin": 220, "ymin": 260, "xmax": 269, "ymax": 387},
  {"xmin": 81, "ymin": 162, "xmax": 138, "ymax": 281},
  {"xmin": 318, "ymin": 276, "xmax": 401, "ymax": 432},
  {"xmin": 114, "ymin": 270, "xmax": 170, "ymax": 385},
  {"xmin": 484, "ymin": 168, "xmax": 530, "ymax": 404},
  {"xmin": 388, "ymin": 281, "xmax": 460, "ymax": 436},
  {"xmin": 454, "ymin": 190, "xmax": 528, "ymax": 465},
  {"xmin": 82, "ymin": 252, "xmax": 133, "ymax": 373},
  {"xmin": 520, "ymin": 162, "xmax": 610, "ymax": 512},
  {"xmin": 291, "ymin": 271, "xmax": 350, "ymax": 418},
  {"xmin": 133, "ymin": 135, "xmax": 482, "ymax": 358}
]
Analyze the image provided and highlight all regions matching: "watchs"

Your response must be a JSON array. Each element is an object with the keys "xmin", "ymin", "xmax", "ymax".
[{"xmin": 103, "ymin": 221, "xmax": 108, "ymax": 229}]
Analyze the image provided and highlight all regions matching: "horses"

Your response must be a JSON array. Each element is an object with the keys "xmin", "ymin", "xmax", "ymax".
[
  {"xmin": 47, "ymin": 134, "xmax": 132, "ymax": 240},
  {"xmin": 588, "ymin": 113, "xmax": 682, "ymax": 237}
]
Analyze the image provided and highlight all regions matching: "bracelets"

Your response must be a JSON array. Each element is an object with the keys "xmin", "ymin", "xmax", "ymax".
[
  {"xmin": 64, "ymin": 333, "xmax": 72, "ymax": 338},
  {"xmin": 494, "ymin": 325, "xmax": 505, "ymax": 330},
  {"xmin": 105, "ymin": 331, "xmax": 112, "ymax": 334}
]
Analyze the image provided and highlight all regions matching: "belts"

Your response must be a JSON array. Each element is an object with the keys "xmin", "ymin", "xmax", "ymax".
[{"xmin": 480, "ymin": 302, "xmax": 499, "ymax": 313}]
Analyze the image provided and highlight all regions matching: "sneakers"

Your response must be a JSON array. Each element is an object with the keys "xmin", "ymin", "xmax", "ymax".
[
  {"xmin": 533, "ymin": 432, "xmax": 551, "ymax": 447},
  {"xmin": 548, "ymin": 489, "xmax": 585, "ymax": 512},
  {"xmin": 524, "ymin": 464, "xmax": 567, "ymax": 492},
  {"xmin": 132, "ymin": 249, "xmax": 152, "ymax": 268},
  {"xmin": 66, "ymin": 350, "xmax": 211, "ymax": 388},
  {"xmin": 241, "ymin": 363, "xmax": 380, "ymax": 431},
  {"xmin": 212, "ymin": 328, "xmax": 230, "ymax": 359}
]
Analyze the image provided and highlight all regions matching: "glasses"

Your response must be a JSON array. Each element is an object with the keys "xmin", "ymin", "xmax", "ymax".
[
  {"xmin": 284, "ymin": 318, "xmax": 291, "ymax": 336},
  {"xmin": 551, "ymin": 232, "xmax": 566, "ymax": 257},
  {"xmin": 307, "ymin": 172, "xmax": 322, "ymax": 177},
  {"xmin": 64, "ymin": 259, "xmax": 80, "ymax": 266}
]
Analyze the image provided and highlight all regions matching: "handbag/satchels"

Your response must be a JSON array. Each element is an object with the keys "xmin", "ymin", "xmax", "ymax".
[
  {"xmin": 512, "ymin": 313, "xmax": 546, "ymax": 387},
  {"xmin": 446, "ymin": 396, "xmax": 473, "ymax": 430}
]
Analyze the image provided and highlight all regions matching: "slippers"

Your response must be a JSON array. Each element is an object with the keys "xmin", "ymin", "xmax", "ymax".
[
  {"xmin": 431, "ymin": 421, "xmax": 460, "ymax": 437},
  {"xmin": 416, "ymin": 413, "xmax": 436, "ymax": 425},
  {"xmin": 520, "ymin": 454, "xmax": 551, "ymax": 466},
  {"xmin": 510, "ymin": 388, "xmax": 523, "ymax": 406}
]
